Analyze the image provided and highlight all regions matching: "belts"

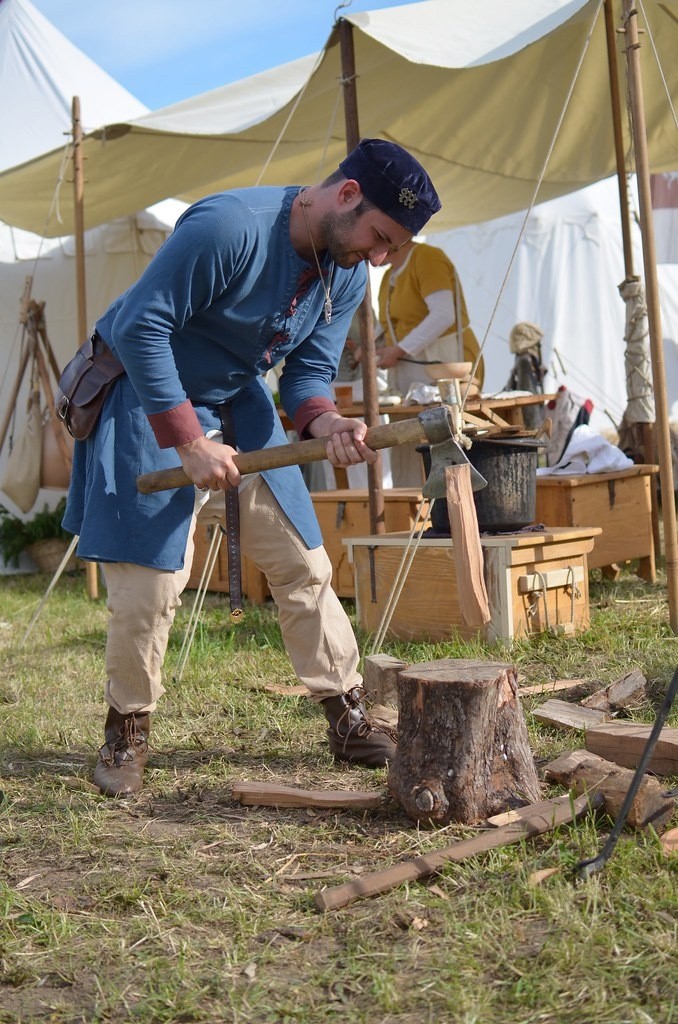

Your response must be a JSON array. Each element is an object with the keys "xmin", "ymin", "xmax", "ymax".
[{"xmin": 194, "ymin": 400, "xmax": 243, "ymax": 624}]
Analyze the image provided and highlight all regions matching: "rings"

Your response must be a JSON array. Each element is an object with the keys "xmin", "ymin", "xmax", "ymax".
[{"xmin": 194, "ymin": 484, "xmax": 209, "ymax": 492}]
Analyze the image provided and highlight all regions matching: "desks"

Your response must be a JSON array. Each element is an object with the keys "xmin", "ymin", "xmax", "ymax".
[{"xmin": 276, "ymin": 394, "xmax": 561, "ymax": 487}]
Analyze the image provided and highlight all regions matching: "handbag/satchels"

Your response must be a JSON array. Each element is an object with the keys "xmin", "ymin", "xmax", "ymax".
[{"xmin": 55, "ymin": 334, "xmax": 125, "ymax": 441}]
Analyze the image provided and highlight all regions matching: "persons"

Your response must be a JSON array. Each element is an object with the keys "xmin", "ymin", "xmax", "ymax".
[
  {"xmin": 61, "ymin": 138, "xmax": 442, "ymax": 799},
  {"xmin": 290, "ymin": 306, "xmax": 393, "ymax": 492},
  {"xmin": 376, "ymin": 240, "xmax": 485, "ymax": 492}
]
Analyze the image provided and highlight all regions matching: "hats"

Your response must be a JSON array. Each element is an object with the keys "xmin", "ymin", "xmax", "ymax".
[{"xmin": 339, "ymin": 138, "xmax": 442, "ymax": 236}]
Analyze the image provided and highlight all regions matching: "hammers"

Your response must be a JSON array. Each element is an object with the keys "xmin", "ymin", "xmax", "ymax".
[{"xmin": 313, "ymin": 757, "xmax": 675, "ymax": 914}]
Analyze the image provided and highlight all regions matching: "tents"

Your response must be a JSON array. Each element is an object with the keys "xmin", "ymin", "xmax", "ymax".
[{"xmin": 0, "ymin": 0, "xmax": 677, "ymax": 634}]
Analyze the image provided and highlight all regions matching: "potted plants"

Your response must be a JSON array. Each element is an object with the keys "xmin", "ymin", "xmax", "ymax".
[{"xmin": 0, "ymin": 496, "xmax": 87, "ymax": 575}]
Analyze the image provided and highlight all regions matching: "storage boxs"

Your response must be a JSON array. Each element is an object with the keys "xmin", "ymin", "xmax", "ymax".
[
  {"xmin": 309, "ymin": 488, "xmax": 434, "ymax": 598},
  {"xmin": 341, "ymin": 527, "xmax": 603, "ymax": 652},
  {"xmin": 185, "ymin": 509, "xmax": 271, "ymax": 606},
  {"xmin": 528, "ymin": 464, "xmax": 660, "ymax": 584}
]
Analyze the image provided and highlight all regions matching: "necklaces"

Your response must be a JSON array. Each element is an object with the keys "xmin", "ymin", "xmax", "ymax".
[{"xmin": 299, "ymin": 188, "xmax": 335, "ymax": 325}]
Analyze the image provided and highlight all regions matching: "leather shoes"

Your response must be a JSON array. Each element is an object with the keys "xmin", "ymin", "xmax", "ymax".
[
  {"xmin": 320, "ymin": 687, "xmax": 398, "ymax": 769},
  {"xmin": 94, "ymin": 707, "xmax": 149, "ymax": 798}
]
